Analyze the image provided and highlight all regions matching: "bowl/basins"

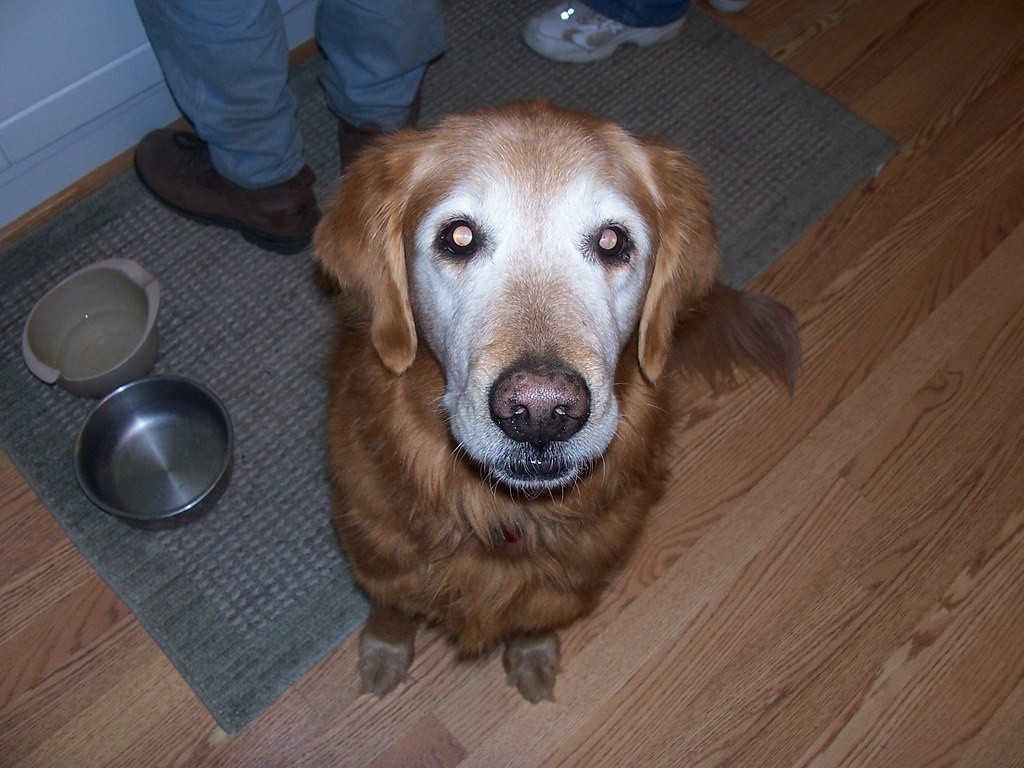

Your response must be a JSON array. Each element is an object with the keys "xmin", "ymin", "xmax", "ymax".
[
  {"xmin": 21, "ymin": 257, "xmax": 161, "ymax": 400},
  {"xmin": 73, "ymin": 374, "xmax": 234, "ymax": 531}
]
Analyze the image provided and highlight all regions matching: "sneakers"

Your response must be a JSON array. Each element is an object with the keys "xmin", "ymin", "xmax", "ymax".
[
  {"xmin": 523, "ymin": 0, "xmax": 688, "ymax": 64},
  {"xmin": 339, "ymin": 50, "xmax": 449, "ymax": 184},
  {"xmin": 707, "ymin": 0, "xmax": 753, "ymax": 13},
  {"xmin": 134, "ymin": 129, "xmax": 325, "ymax": 256}
]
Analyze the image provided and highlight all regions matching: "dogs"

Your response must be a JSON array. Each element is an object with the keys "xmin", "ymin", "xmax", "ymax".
[{"xmin": 308, "ymin": 96, "xmax": 802, "ymax": 708}]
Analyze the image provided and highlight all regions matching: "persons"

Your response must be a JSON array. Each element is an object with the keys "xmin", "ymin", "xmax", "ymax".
[
  {"xmin": 521, "ymin": 0, "xmax": 753, "ymax": 64},
  {"xmin": 130, "ymin": 0, "xmax": 448, "ymax": 259}
]
